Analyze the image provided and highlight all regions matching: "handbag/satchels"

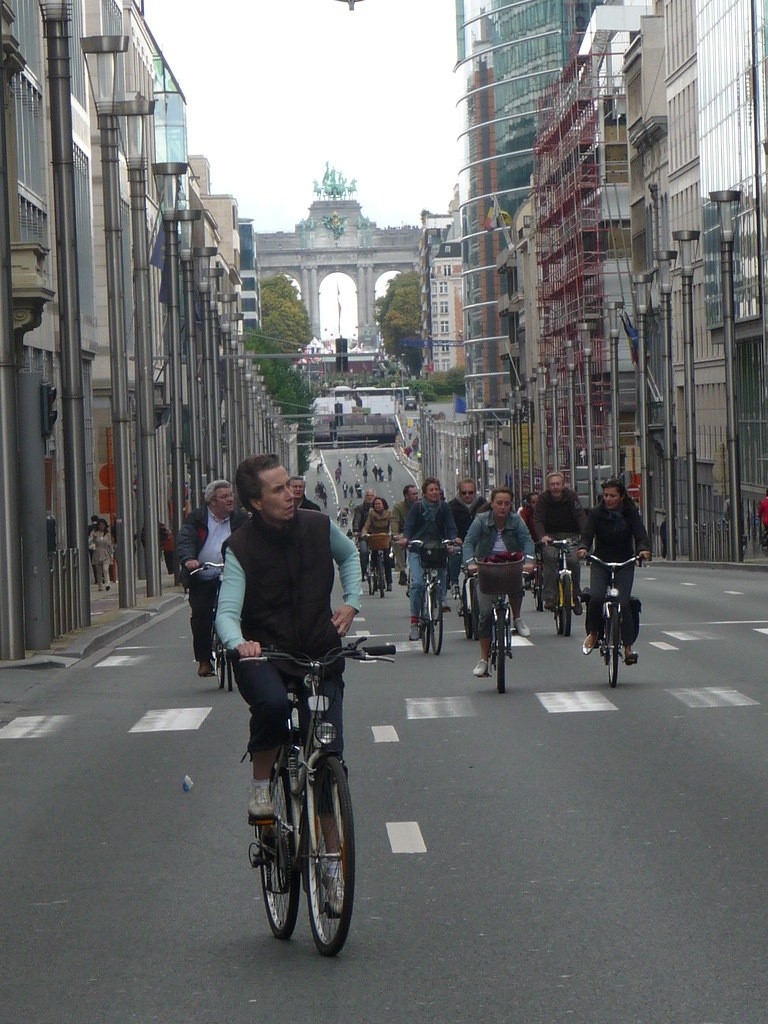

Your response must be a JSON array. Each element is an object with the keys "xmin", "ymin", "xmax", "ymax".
[{"xmin": 88, "ymin": 541, "xmax": 95, "ymax": 551}]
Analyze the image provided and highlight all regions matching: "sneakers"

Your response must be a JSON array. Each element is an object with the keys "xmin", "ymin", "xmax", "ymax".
[
  {"xmin": 512, "ymin": 617, "xmax": 531, "ymax": 637},
  {"xmin": 246, "ymin": 782, "xmax": 274, "ymax": 819},
  {"xmin": 324, "ymin": 866, "xmax": 345, "ymax": 914},
  {"xmin": 437, "ymin": 600, "xmax": 451, "ymax": 614},
  {"xmin": 408, "ymin": 623, "xmax": 420, "ymax": 641},
  {"xmin": 472, "ymin": 658, "xmax": 490, "ymax": 677}
]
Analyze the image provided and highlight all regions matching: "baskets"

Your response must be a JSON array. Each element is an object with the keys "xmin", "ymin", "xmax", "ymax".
[
  {"xmin": 419, "ymin": 546, "xmax": 449, "ymax": 569},
  {"xmin": 473, "ymin": 554, "xmax": 523, "ymax": 595},
  {"xmin": 367, "ymin": 533, "xmax": 390, "ymax": 551}
]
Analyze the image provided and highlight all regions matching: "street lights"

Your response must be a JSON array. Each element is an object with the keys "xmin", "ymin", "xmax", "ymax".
[
  {"xmin": 417, "ymin": 250, "xmax": 678, "ymax": 560},
  {"xmin": 115, "ymin": 98, "xmax": 163, "ymax": 608},
  {"xmin": 709, "ymin": 188, "xmax": 741, "ymax": 562},
  {"xmin": 150, "ymin": 162, "xmax": 293, "ymax": 588},
  {"xmin": 73, "ymin": 34, "xmax": 135, "ymax": 627},
  {"xmin": 671, "ymin": 229, "xmax": 699, "ymax": 562}
]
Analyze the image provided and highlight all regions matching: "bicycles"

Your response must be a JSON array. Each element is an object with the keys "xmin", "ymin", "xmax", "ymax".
[
  {"xmin": 346, "ymin": 529, "xmax": 580, "ymax": 694},
  {"xmin": 190, "ymin": 562, "xmax": 233, "ymax": 692},
  {"xmin": 323, "ymin": 499, "xmax": 327, "ymax": 508},
  {"xmin": 341, "ymin": 517, "xmax": 346, "ymax": 528},
  {"xmin": 225, "ymin": 636, "xmax": 396, "ymax": 958},
  {"xmin": 586, "ymin": 553, "xmax": 638, "ymax": 687}
]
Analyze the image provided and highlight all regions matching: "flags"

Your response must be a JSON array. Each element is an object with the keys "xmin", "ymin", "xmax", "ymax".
[{"xmin": 455, "ymin": 397, "xmax": 466, "ymax": 413}]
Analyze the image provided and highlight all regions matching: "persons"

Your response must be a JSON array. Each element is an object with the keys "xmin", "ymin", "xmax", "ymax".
[
  {"xmin": 363, "ymin": 453, "xmax": 367, "ymax": 466},
  {"xmin": 356, "ymin": 454, "xmax": 359, "ymax": 466},
  {"xmin": 447, "ymin": 479, "xmax": 487, "ymax": 599},
  {"xmin": 353, "ymin": 488, "xmax": 377, "ymax": 582},
  {"xmin": 757, "ymin": 488, "xmax": 768, "ymax": 553},
  {"xmin": 372, "ymin": 465, "xmax": 384, "ymax": 481},
  {"xmin": 336, "ymin": 499, "xmax": 355, "ymax": 528},
  {"xmin": 335, "ymin": 459, "xmax": 341, "ymax": 481},
  {"xmin": 577, "ymin": 480, "xmax": 650, "ymax": 665},
  {"xmin": 363, "ymin": 468, "xmax": 367, "ymax": 482},
  {"xmin": 289, "ymin": 476, "xmax": 321, "ymax": 511},
  {"xmin": 88, "ymin": 515, "xmax": 171, "ymax": 591},
  {"xmin": 314, "ymin": 481, "xmax": 327, "ymax": 509},
  {"xmin": 214, "ymin": 454, "xmax": 363, "ymax": 917},
  {"xmin": 388, "ymin": 464, "xmax": 392, "ymax": 480},
  {"xmin": 519, "ymin": 493, "xmax": 541, "ymax": 542},
  {"xmin": 400, "ymin": 477, "xmax": 462, "ymax": 641},
  {"xmin": 660, "ymin": 518, "xmax": 678, "ymax": 558},
  {"xmin": 461, "ymin": 486, "xmax": 535, "ymax": 676},
  {"xmin": 174, "ymin": 480, "xmax": 251, "ymax": 677},
  {"xmin": 360, "ymin": 497, "xmax": 393, "ymax": 591},
  {"xmin": 343, "ymin": 481, "xmax": 362, "ymax": 498},
  {"xmin": 533, "ymin": 471, "xmax": 588, "ymax": 615},
  {"xmin": 390, "ymin": 485, "xmax": 422, "ymax": 586}
]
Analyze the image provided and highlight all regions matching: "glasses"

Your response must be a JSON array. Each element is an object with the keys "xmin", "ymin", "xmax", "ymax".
[
  {"xmin": 460, "ymin": 490, "xmax": 476, "ymax": 495},
  {"xmin": 213, "ymin": 492, "xmax": 235, "ymax": 500}
]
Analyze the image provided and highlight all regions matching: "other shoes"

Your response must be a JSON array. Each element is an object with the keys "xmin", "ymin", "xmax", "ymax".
[
  {"xmin": 625, "ymin": 651, "xmax": 639, "ymax": 666},
  {"xmin": 95, "ymin": 578, "xmax": 111, "ymax": 591},
  {"xmin": 361, "ymin": 568, "xmax": 393, "ymax": 592},
  {"xmin": 582, "ymin": 630, "xmax": 599, "ymax": 655},
  {"xmin": 398, "ymin": 573, "xmax": 407, "ymax": 586},
  {"xmin": 197, "ymin": 659, "xmax": 215, "ymax": 677}
]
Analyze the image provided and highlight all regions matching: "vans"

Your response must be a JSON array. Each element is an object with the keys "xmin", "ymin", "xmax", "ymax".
[{"xmin": 404, "ymin": 398, "xmax": 416, "ymax": 411}]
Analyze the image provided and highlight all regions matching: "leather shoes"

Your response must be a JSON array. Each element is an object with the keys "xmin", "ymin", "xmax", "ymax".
[
  {"xmin": 573, "ymin": 597, "xmax": 584, "ymax": 616},
  {"xmin": 544, "ymin": 597, "xmax": 555, "ymax": 610}
]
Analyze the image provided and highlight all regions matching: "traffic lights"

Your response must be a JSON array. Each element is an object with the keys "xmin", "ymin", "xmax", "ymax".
[{"xmin": 39, "ymin": 381, "xmax": 58, "ymax": 436}]
